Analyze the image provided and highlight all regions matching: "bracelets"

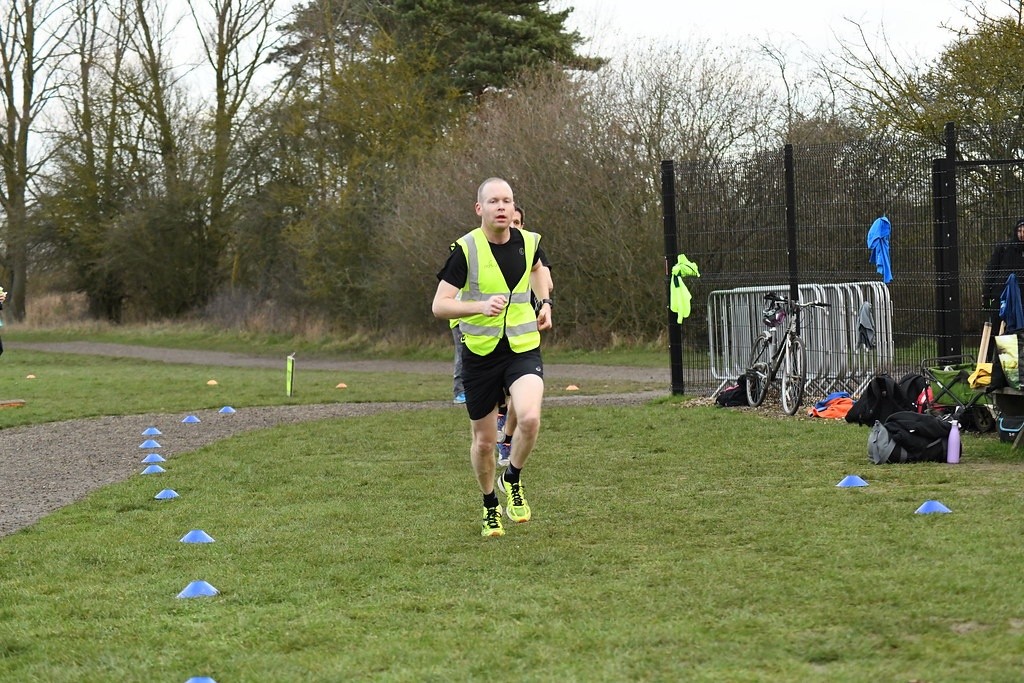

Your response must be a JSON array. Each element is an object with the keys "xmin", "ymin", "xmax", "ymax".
[{"xmin": 537, "ymin": 298, "xmax": 553, "ymax": 310}]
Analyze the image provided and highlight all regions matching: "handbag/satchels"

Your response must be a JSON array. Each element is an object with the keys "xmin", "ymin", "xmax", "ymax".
[
  {"xmin": 867, "ymin": 414, "xmax": 962, "ymax": 463},
  {"xmin": 998, "ymin": 415, "xmax": 1024, "ymax": 443}
]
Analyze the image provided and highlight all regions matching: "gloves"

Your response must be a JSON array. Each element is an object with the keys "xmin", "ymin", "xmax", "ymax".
[{"xmin": 980, "ymin": 292, "xmax": 996, "ymax": 309}]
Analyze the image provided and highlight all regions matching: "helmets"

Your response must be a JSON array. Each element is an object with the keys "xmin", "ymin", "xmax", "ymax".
[{"xmin": 763, "ymin": 307, "xmax": 785, "ymax": 328}]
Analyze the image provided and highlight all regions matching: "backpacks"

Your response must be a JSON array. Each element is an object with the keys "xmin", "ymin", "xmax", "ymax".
[
  {"xmin": 897, "ymin": 374, "xmax": 933, "ymax": 418},
  {"xmin": 863, "ymin": 375, "xmax": 911, "ymax": 427}
]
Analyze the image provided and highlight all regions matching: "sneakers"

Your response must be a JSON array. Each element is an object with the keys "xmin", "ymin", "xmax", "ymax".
[
  {"xmin": 496, "ymin": 472, "xmax": 532, "ymax": 522},
  {"xmin": 496, "ymin": 413, "xmax": 506, "ymax": 434},
  {"xmin": 481, "ymin": 504, "xmax": 505, "ymax": 538},
  {"xmin": 496, "ymin": 442, "xmax": 511, "ymax": 466}
]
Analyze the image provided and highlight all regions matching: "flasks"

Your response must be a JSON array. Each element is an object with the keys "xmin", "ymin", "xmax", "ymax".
[{"xmin": 947, "ymin": 420, "xmax": 961, "ymax": 464}]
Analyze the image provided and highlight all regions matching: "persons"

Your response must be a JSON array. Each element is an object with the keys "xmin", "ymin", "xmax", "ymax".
[
  {"xmin": 981, "ymin": 220, "xmax": 1024, "ymax": 363},
  {"xmin": 497, "ymin": 205, "xmax": 554, "ymax": 467},
  {"xmin": 447, "ymin": 292, "xmax": 467, "ymax": 403},
  {"xmin": 432, "ymin": 177, "xmax": 553, "ymax": 538}
]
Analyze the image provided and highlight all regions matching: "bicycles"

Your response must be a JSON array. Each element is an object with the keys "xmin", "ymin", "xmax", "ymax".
[{"xmin": 745, "ymin": 291, "xmax": 831, "ymax": 416}]
